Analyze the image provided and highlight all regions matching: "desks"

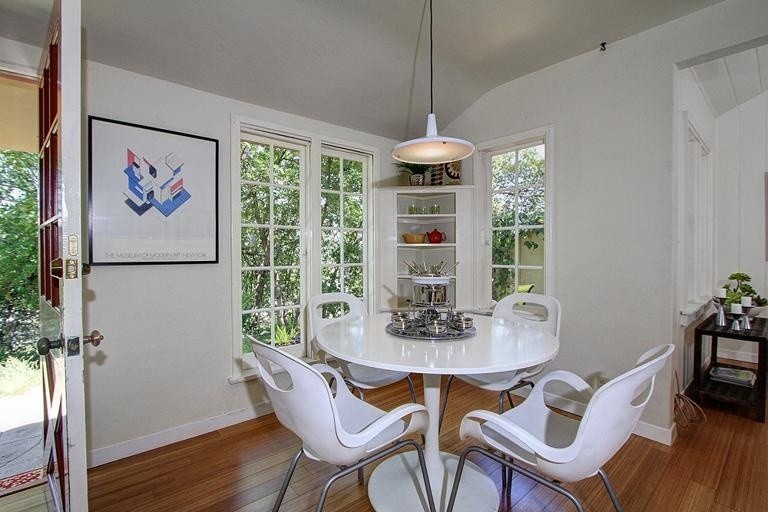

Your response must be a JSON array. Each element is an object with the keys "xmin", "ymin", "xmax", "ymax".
[
  {"xmin": 693, "ymin": 313, "xmax": 767, "ymax": 422},
  {"xmin": 316, "ymin": 314, "xmax": 560, "ymax": 512}
]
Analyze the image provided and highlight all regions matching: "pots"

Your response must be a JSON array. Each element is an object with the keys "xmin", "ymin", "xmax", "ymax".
[{"xmin": 412, "ymin": 283, "xmax": 447, "ymax": 304}]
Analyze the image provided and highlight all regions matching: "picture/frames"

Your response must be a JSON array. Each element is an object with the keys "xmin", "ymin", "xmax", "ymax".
[{"xmin": 88, "ymin": 115, "xmax": 219, "ymax": 265}]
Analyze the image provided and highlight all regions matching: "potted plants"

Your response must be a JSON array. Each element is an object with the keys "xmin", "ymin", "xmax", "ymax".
[
  {"xmin": 392, "ymin": 161, "xmax": 429, "ymax": 186},
  {"xmin": 712, "ymin": 272, "xmax": 756, "ymax": 319}
]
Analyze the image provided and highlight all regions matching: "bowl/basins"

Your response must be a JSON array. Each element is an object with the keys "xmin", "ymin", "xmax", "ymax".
[
  {"xmin": 390, "ymin": 310, "xmax": 474, "ymax": 334},
  {"xmin": 402, "ymin": 233, "xmax": 426, "ymax": 244}
]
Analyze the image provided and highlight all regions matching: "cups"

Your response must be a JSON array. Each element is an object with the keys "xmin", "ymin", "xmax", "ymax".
[{"xmin": 407, "ymin": 200, "xmax": 441, "ymax": 214}]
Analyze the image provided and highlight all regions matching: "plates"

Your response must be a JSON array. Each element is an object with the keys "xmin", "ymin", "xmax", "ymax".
[{"xmin": 385, "ymin": 320, "xmax": 477, "ymax": 341}]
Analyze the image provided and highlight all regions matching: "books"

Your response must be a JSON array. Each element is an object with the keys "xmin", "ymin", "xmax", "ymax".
[{"xmin": 708, "ymin": 366, "xmax": 757, "ymax": 390}]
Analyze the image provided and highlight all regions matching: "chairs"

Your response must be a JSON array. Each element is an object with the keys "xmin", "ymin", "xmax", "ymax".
[
  {"xmin": 246, "ymin": 333, "xmax": 436, "ymax": 512},
  {"xmin": 305, "ymin": 291, "xmax": 425, "ymax": 445},
  {"xmin": 446, "ymin": 344, "xmax": 676, "ymax": 512},
  {"xmin": 439, "ymin": 292, "xmax": 561, "ymax": 490}
]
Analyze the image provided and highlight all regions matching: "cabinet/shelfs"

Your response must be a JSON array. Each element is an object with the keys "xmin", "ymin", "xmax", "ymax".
[{"xmin": 393, "ymin": 190, "xmax": 458, "ymax": 312}]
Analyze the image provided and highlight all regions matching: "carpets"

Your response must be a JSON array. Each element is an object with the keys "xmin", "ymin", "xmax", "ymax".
[{"xmin": 0, "ymin": 421, "xmax": 47, "ymax": 498}]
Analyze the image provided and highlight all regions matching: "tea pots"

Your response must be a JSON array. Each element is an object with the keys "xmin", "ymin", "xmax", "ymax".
[{"xmin": 426, "ymin": 228, "xmax": 447, "ymax": 243}]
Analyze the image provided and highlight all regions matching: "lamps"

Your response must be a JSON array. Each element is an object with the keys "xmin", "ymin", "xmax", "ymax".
[{"xmin": 390, "ymin": 0, "xmax": 475, "ymax": 165}]
